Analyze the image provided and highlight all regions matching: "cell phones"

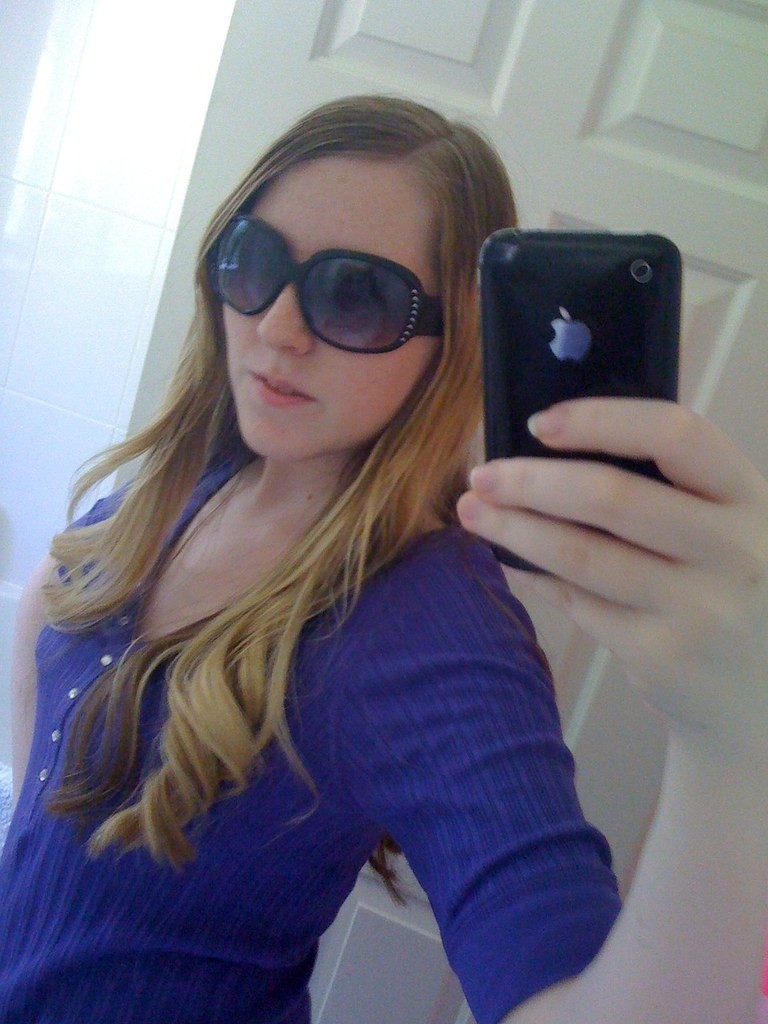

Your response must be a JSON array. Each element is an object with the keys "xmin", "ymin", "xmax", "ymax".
[{"xmin": 476, "ymin": 229, "xmax": 684, "ymax": 580}]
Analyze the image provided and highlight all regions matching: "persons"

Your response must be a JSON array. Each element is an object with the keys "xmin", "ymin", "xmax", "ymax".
[{"xmin": 0, "ymin": 92, "xmax": 768, "ymax": 1024}]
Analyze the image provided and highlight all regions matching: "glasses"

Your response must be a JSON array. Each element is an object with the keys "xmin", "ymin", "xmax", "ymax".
[{"xmin": 204, "ymin": 214, "xmax": 446, "ymax": 353}]
